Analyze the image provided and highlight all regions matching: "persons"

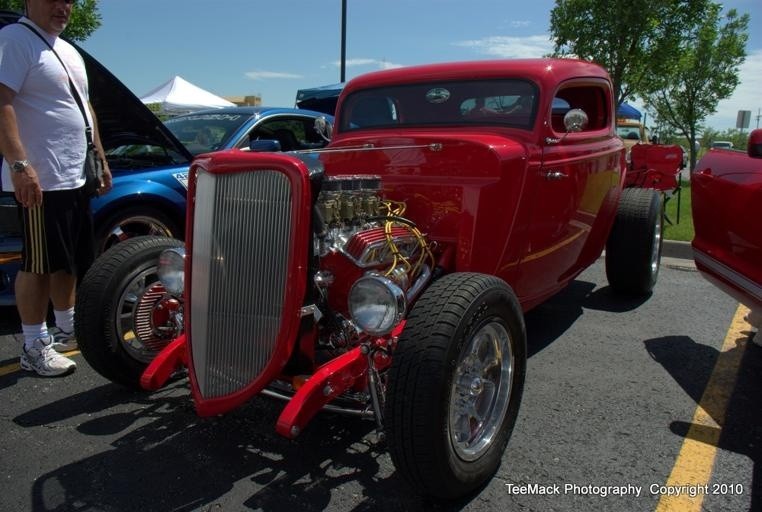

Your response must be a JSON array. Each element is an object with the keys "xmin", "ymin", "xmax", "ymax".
[{"xmin": 1, "ymin": 0, "xmax": 112, "ymax": 378}]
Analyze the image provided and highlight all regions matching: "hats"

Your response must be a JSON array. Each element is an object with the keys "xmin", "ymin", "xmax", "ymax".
[{"xmin": 66, "ymin": 55, "xmax": 689, "ymax": 501}]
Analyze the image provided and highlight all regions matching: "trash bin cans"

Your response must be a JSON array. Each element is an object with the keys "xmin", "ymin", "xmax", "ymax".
[{"xmin": 9, "ymin": 161, "xmax": 31, "ymax": 172}]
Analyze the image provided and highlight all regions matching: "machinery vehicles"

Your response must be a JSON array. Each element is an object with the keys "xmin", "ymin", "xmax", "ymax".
[{"xmin": 1, "ymin": 6, "xmax": 365, "ymax": 312}]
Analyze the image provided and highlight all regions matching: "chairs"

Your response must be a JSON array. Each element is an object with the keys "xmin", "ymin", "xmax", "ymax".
[{"xmin": 20, "ymin": 328, "xmax": 77, "ymax": 377}]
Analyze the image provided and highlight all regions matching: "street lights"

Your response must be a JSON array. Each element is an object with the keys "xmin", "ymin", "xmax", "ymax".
[{"xmin": 85, "ymin": 144, "xmax": 104, "ymax": 193}]
